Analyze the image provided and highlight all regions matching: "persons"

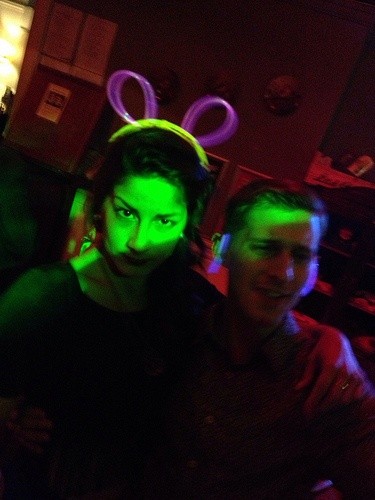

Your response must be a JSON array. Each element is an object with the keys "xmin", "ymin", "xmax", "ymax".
[
  {"xmin": 137, "ymin": 178, "xmax": 374, "ymax": 500},
  {"xmin": 0, "ymin": 128, "xmax": 370, "ymax": 500}
]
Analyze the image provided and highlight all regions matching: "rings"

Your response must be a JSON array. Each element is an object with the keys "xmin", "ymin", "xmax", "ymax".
[{"xmin": 337, "ymin": 376, "xmax": 350, "ymax": 392}]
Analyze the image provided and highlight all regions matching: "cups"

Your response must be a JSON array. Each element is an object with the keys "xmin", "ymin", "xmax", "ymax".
[{"xmin": 50, "ymin": 442, "xmax": 101, "ymax": 500}]
